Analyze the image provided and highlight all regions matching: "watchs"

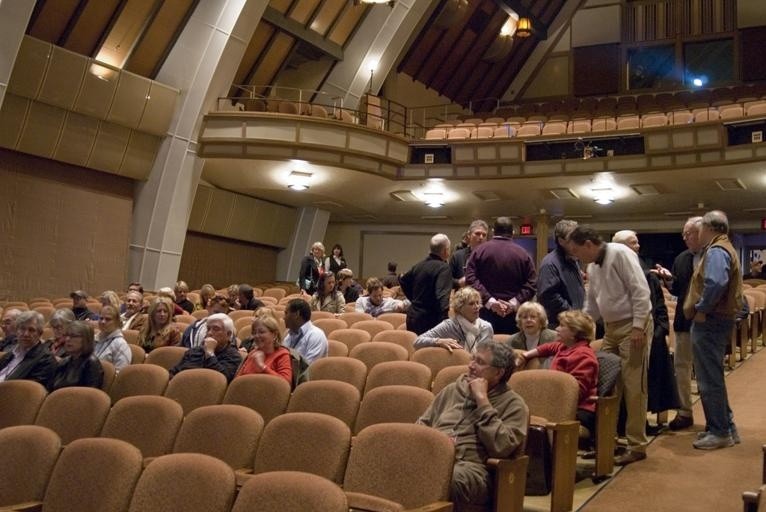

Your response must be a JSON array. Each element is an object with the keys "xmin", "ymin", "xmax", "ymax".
[{"xmin": 205, "ymin": 351, "xmax": 215, "ymax": 359}]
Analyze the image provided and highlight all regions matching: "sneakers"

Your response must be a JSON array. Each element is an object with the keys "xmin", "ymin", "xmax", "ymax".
[
  {"xmin": 580, "ymin": 449, "xmax": 595, "ymax": 459},
  {"xmin": 668, "ymin": 414, "xmax": 695, "ymax": 431},
  {"xmin": 694, "ymin": 434, "xmax": 736, "ymax": 450},
  {"xmin": 697, "ymin": 427, "xmax": 742, "ymax": 444},
  {"xmin": 613, "ymin": 450, "xmax": 648, "ymax": 465}
]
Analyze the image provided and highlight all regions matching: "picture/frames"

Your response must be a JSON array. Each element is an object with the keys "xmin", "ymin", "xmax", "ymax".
[{"xmin": 0, "ymin": 379, "xmax": 46, "ymax": 430}]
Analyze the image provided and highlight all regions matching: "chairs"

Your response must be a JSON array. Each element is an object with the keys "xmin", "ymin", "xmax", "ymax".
[
  {"xmin": 128, "ymin": 454, "xmax": 236, "ymax": 512},
  {"xmin": 426, "ymin": 84, "xmax": 766, "ymax": 139},
  {"xmin": 164, "ymin": 369, "xmax": 225, "ymax": 408},
  {"xmin": 34, "ymin": 387, "xmax": 112, "ymax": 444},
  {"xmin": 411, "ymin": 347, "xmax": 472, "ymax": 366},
  {"xmin": 124, "ymin": 327, "xmax": 142, "ymax": 345},
  {"xmin": 240, "ymin": 281, "xmax": 313, "ymax": 307},
  {"xmin": 367, "ymin": 360, "xmax": 432, "ymax": 392},
  {"xmin": 231, "ymin": 470, "xmax": 347, "ymax": 512},
  {"xmin": 356, "ymin": 385, "xmax": 435, "ymax": 433},
  {"xmin": 508, "ymin": 369, "xmax": 578, "ymax": 512},
  {"xmin": 0, "ymin": 425, "xmax": 60, "ymax": 512},
  {"xmin": 222, "ymin": 374, "xmax": 290, "ymax": 418},
  {"xmin": 127, "ymin": 344, "xmax": 147, "ymax": 362},
  {"xmin": 99, "ymin": 395, "xmax": 183, "ymax": 455},
  {"xmin": 342, "ymin": 423, "xmax": 455, "ymax": 510},
  {"xmin": 110, "ymin": 363, "xmax": 170, "ymax": 402},
  {"xmin": 42, "ymin": 438, "xmax": 142, "ymax": 512},
  {"xmin": 147, "ymin": 347, "xmax": 188, "ymax": 368},
  {"xmin": 236, "ymin": 412, "xmax": 351, "ymax": 487},
  {"xmin": 139, "ymin": 404, "xmax": 265, "ymax": 467},
  {"xmin": 312, "ymin": 313, "xmax": 417, "ymax": 344},
  {"xmin": 309, "ymin": 356, "xmax": 366, "ymax": 398},
  {"xmin": 349, "ymin": 341, "xmax": 410, "ymax": 368},
  {"xmin": 267, "ymin": 99, "xmax": 354, "ymax": 121},
  {"xmin": 488, "ymin": 393, "xmax": 530, "ymax": 511},
  {"xmin": 227, "ymin": 309, "xmax": 256, "ymax": 335},
  {"xmin": 430, "ymin": 364, "xmax": 469, "ymax": 392},
  {"xmin": 741, "ymin": 278, "xmax": 766, "ymax": 358},
  {"xmin": 286, "ymin": 380, "xmax": 359, "ymax": 425}
]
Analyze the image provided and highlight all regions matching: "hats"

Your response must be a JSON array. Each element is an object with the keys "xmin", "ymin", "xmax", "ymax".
[{"xmin": 70, "ymin": 290, "xmax": 89, "ymax": 299}]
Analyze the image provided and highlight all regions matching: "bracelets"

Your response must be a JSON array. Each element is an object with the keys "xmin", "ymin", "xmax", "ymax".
[{"xmin": 261, "ymin": 365, "xmax": 267, "ymax": 373}]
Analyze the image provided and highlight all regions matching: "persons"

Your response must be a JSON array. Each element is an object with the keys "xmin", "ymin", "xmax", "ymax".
[
  {"xmin": 399, "ymin": 233, "xmax": 452, "ymax": 336},
  {"xmin": 0, "ymin": 308, "xmax": 22, "ymax": 371},
  {"xmin": 159, "ymin": 287, "xmax": 183, "ymax": 315},
  {"xmin": 70, "ymin": 290, "xmax": 93, "ymax": 320},
  {"xmin": 683, "ymin": 210, "xmax": 744, "ymax": 450},
  {"xmin": 414, "ymin": 341, "xmax": 529, "ymax": 511},
  {"xmin": 649, "ymin": 216, "xmax": 704, "ymax": 431},
  {"xmin": 501, "ymin": 301, "xmax": 558, "ymax": 350},
  {"xmin": 335, "ymin": 268, "xmax": 364, "ymax": 304},
  {"xmin": 192, "ymin": 284, "xmax": 216, "ymax": 313},
  {"xmin": 566, "ymin": 224, "xmax": 653, "ymax": 466},
  {"xmin": 612, "ymin": 230, "xmax": 682, "ymax": 436},
  {"xmin": 43, "ymin": 308, "xmax": 76, "ymax": 364},
  {"xmin": 120, "ymin": 291, "xmax": 148, "ymax": 331},
  {"xmin": 382, "ymin": 262, "xmax": 400, "ymax": 289},
  {"xmin": 536, "ymin": 220, "xmax": 589, "ymax": 331},
  {"xmin": 281, "ymin": 298, "xmax": 328, "ymax": 366},
  {"xmin": 390, "ymin": 272, "xmax": 409, "ymax": 301},
  {"xmin": 180, "ymin": 294, "xmax": 236, "ymax": 350},
  {"xmin": 299, "ymin": 242, "xmax": 325, "ymax": 296},
  {"xmin": 226, "ymin": 284, "xmax": 241, "ymax": 311},
  {"xmin": 325, "ymin": 244, "xmax": 346, "ymax": 274},
  {"xmin": 174, "ymin": 281, "xmax": 194, "ymax": 315},
  {"xmin": 355, "ymin": 278, "xmax": 412, "ymax": 317},
  {"xmin": 517, "ymin": 310, "xmax": 599, "ymax": 432},
  {"xmin": 306, "ymin": 272, "xmax": 345, "ymax": 314},
  {"xmin": 465, "ymin": 216, "xmax": 538, "ymax": 335},
  {"xmin": 413, "ymin": 286, "xmax": 494, "ymax": 354},
  {"xmin": 238, "ymin": 284, "xmax": 266, "ymax": 310},
  {"xmin": 233, "ymin": 318, "xmax": 292, "ymax": 386},
  {"xmin": 169, "ymin": 313, "xmax": 242, "ymax": 385},
  {"xmin": 120, "ymin": 282, "xmax": 144, "ymax": 314},
  {"xmin": 92, "ymin": 305, "xmax": 133, "ymax": 377},
  {"xmin": 449, "ymin": 219, "xmax": 488, "ymax": 292},
  {"xmin": 0, "ymin": 310, "xmax": 59, "ymax": 391},
  {"xmin": 238, "ymin": 307, "xmax": 279, "ymax": 354},
  {"xmin": 46, "ymin": 322, "xmax": 104, "ymax": 394},
  {"xmin": 92, "ymin": 291, "xmax": 122, "ymax": 320},
  {"xmin": 135, "ymin": 298, "xmax": 182, "ymax": 358}
]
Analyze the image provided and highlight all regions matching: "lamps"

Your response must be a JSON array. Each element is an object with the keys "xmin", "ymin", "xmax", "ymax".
[{"xmin": 516, "ymin": 4, "xmax": 533, "ymax": 37}]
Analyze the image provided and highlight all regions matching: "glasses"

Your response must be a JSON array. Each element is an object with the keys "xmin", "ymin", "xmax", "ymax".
[
  {"xmin": 682, "ymin": 229, "xmax": 701, "ymax": 238},
  {"xmin": 50, "ymin": 324, "xmax": 65, "ymax": 331},
  {"xmin": 62, "ymin": 332, "xmax": 83, "ymax": 339},
  {"xmin": 467, "ymin": 354, "xmax": 505, "ymax": 371}
]
